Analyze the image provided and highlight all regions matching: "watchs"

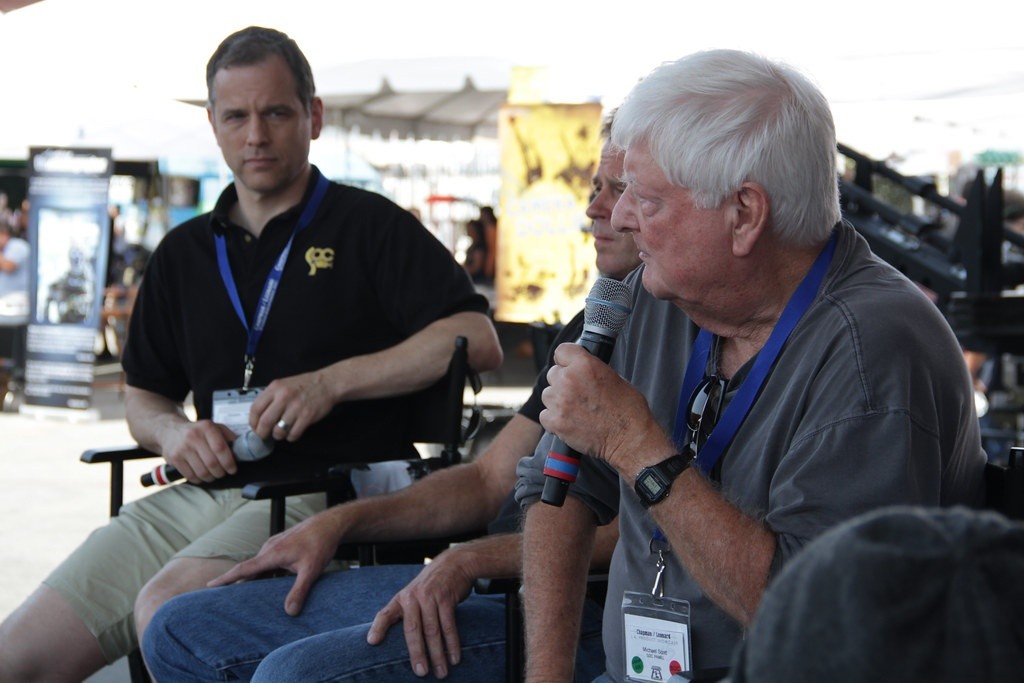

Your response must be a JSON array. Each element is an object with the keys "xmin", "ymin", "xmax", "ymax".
[{"xmin": 633, "ymin": 453, "xmax": 690, "ymax": 508}]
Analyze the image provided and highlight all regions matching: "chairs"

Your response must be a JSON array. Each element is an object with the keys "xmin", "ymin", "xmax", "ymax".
[{"xmin": 80, "ymin": 337, "xmax": 1024, "ymax": 683}]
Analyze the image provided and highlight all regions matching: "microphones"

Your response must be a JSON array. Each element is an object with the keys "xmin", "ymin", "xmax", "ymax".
[
  {"xmin": 537, "ymin": 275, "xmax": 632, "ymax": 510},
  {"xmin": 139, "ymin": 426, "xmax": 275, "ymax": 489}
]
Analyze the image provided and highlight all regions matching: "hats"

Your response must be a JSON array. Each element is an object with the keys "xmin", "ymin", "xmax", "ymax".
[{"xmin": 732, "ymin": 506, "xmax": 1023, "ymax": 683}]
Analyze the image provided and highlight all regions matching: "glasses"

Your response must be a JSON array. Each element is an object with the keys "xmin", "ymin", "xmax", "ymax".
[{"xmin": 681, "ymin": 375, "xmax": 729, "ymax": 458}]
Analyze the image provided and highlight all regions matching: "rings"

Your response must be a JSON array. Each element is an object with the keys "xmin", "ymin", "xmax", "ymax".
[{"xmin": 277, "ymin": 420, "xmax": 290, "ymax": 432}]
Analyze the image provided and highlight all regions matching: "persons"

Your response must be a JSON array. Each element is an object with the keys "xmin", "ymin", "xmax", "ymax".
[
  {"xmin": 515, "ymin": 49, "xmax": 1024, "ymax": 683},
  {"xmin": 0, "ymin": 220, "xmax": 32, "ymax": 412},
  {"xmin": 0, "ymin": 188, "xmax": 152, "ymax": 366},
  {"xmin": 143, "ymin": 105, "xmax": 639, "ymax": 683},
  {"xmin": 0, "ymin": 28, "xmax": 503, "ymax": 683},
  {"xmin": 409, "ymin": 206, "xmax": 497, "ymax": 289}
]
